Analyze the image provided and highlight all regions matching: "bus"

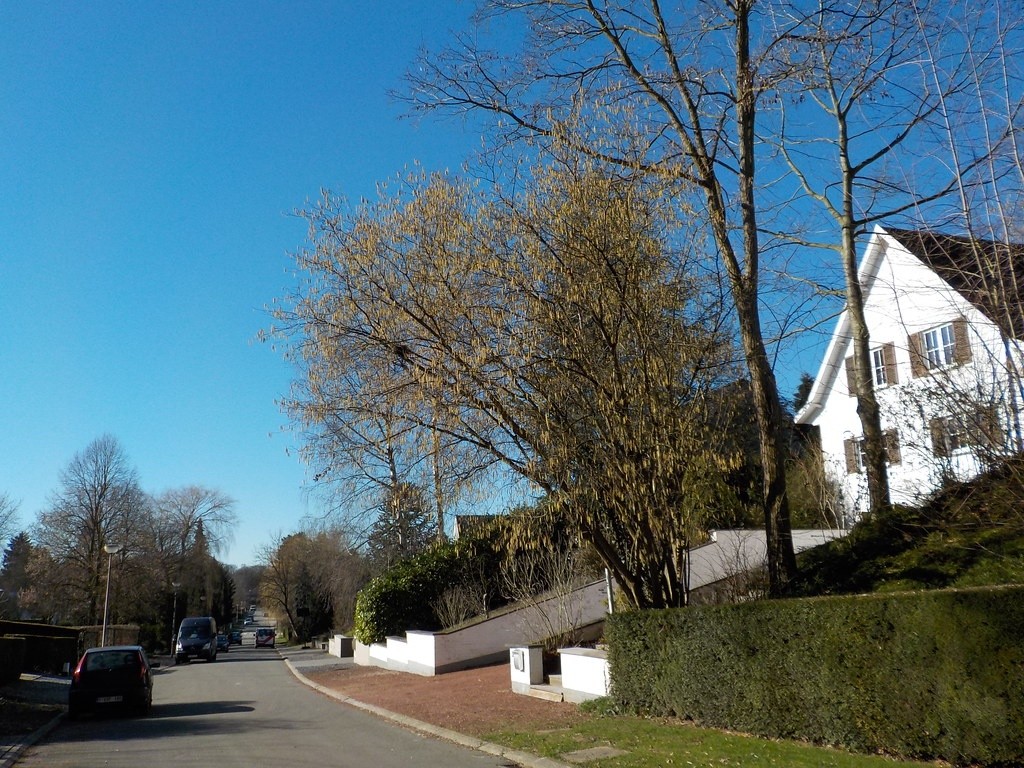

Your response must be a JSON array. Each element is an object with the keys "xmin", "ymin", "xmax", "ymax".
[{"xmin": 253, "ymin": 627, "xmax": 277, "ymax": 649}]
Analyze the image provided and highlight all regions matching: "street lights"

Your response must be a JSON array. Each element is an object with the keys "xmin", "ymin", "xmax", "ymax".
[
  {"xmin": 169, "ymin": 581, "xmax": 182, "ymax": 658},
  {"xmin": 99, "ymin": 543, "xmax": 121, "ymax": 648},
  {"xmin": 200, "ymin": 594, "xmax": 207, "ymax": 617},
  {"xmin": 235, "ymin": 603, "xmax": 239, "ymax": 623}
]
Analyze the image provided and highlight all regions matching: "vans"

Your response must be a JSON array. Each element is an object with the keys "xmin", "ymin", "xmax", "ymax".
[{"xmin": 171, "ymin": 616, "xmax": 219, "ymax": 664}]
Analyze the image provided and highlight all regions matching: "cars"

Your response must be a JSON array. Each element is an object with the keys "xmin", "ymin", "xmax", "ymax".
[
  {"xmin": 243, "ymin": 604, "xmax": 257, "ymax": 626},
  {"xmin": 228, "ymin": 631, "xmax": 242, "ymax": 645},
  {"xmin": 218, "ymin": 634, "xmax": 230, "ymax": 653},
  {"xmin": 68, "ymin": 645, "xmax": 160, "ymax": 718}
]
